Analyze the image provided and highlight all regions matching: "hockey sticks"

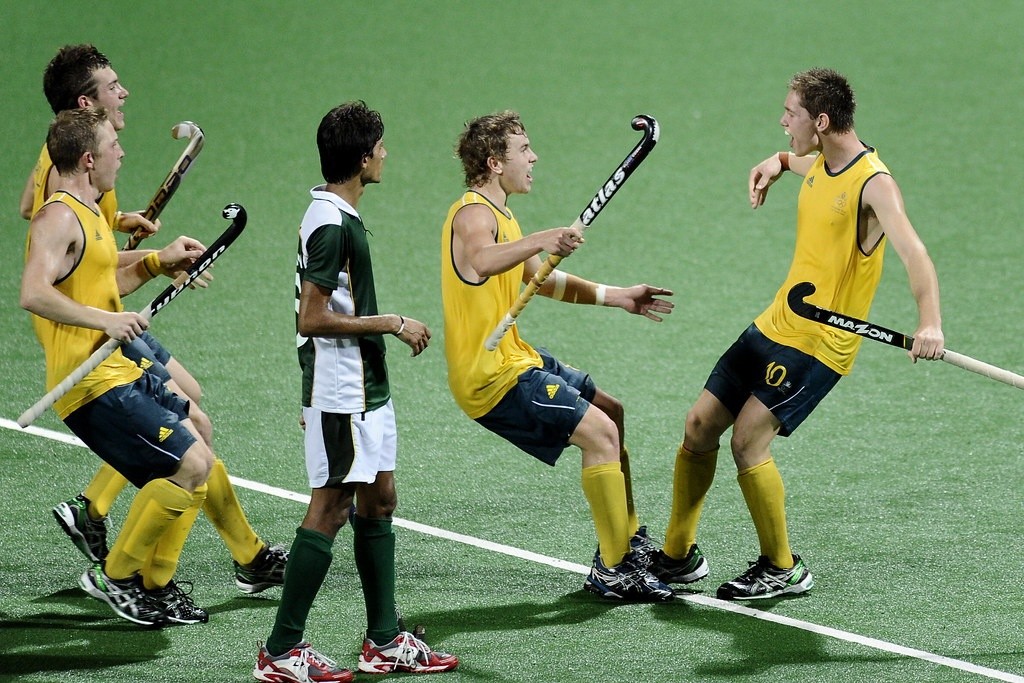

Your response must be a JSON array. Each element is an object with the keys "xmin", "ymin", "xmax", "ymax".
[
  {"xmin": 486, "ymin": 114, "xmax": 662, "ymax": 353},
  {"xmin": 121, "ymin": 120, "xmax": 205, "ymax": 250},
  {"xmin": 788, "ymin": 282, "xmax": 1024, "ymax": 391},
  {"xmin": 16, "ymin": 203, "xmax": 249, "ymax": 428}
]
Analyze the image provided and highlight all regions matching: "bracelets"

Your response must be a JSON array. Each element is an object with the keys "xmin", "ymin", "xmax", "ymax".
[
  {"xmin": 114, "ymin": 210, "xmax": 123, "ymax": 232},
  {"xmin": 395, "ymin": 314, "xmax": 405, "ymax": 337},
  {"xmin": 141, "ymin": 251, "xmax": 165, "ymax": 279}
]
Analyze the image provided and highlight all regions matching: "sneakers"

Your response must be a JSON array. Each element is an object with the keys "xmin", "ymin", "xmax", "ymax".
[
  {"xmin": 236, "ymin": 542, "xmax": 291, "ymax": 593},
  {"xmin": 77, "ymin": 560, "xmax": 167, "ymax": 625},
  {"xmin": 255, "ymin": 638, "xmax": 354, "ymax": 683},
  {"xmin": 584, "ymin": 553, "xmax": 675, "ymax": 602},
  {"xmin": 647, "ymin": 543, "xmax": 710, "ymax": 586},
  {"xmin": 52, "ymin": 491, "xmax": 109, "ymax": 562},
  {"xmin": 716, "ymin": 553, "xmax": 814, "ymax": 600},
  {"xmin": 357, "ymin": 618, "xmax": 460, "ymax": 674},
  {"xmin": 137, "ymin": 575, "xmax": 210, "ymax": 625},
  {"xmin": 594, "ymin": 526, "xmax": 658, "ymax": 572}
]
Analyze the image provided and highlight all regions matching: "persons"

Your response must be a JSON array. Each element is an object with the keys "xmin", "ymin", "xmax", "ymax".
[
  {"xmin": 16, "ymin": 109, "xmax": 221, "ymax": 633},
  {"xmin": 639, "ymin": 65, "xmax": 944, "ymax": 599},
  {"xmin": 444, "ymin": 110, "xmax": 676, "ymax": 605},
  {"xmin": 21, "ymin": 44, "xmax": 292, "ymax": 593},
  {"xmin": 248, "ymin": 101, "xmax": 460, "ymax": 683}
]
